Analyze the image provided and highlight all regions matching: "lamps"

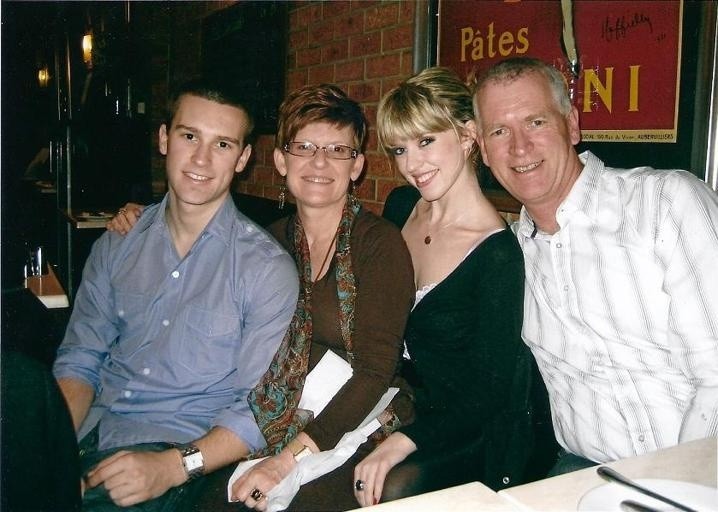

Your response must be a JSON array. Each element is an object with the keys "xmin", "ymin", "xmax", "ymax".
[{"xmin": 82, "ymin": 34, "xmax": 93, "ymax": 66}]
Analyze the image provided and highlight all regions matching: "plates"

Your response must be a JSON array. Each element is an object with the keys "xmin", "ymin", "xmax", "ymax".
[{"xmin": 580, "ymin": 478, "xmax": 717, "ymax": 512}]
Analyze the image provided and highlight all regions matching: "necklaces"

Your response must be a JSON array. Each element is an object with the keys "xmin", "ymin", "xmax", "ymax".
[
  {"xmin": 309, "ymin": 226, "xmax": 337, "ymax": 290},
  {"xmin": 422, "ymin": 210, "xmax": 465, "ymax": 244}
]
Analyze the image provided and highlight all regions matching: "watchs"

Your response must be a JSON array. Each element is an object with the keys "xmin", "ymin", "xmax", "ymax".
[
  {"xmin": 288, "ymin": 437, "xmax": 313, "ymax": 466},
  {"xmin": 177, "ymin": 441, "xmax": 206, "ymax": 485}
]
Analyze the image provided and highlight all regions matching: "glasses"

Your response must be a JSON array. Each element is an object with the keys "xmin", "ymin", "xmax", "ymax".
[{"xmin": 281, "ymin": 140, "xmax": 359, "ymax": 161}]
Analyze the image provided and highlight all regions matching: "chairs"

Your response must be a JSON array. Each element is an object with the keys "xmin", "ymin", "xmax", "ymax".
[{"xmin": 227, "ymin": 189, "xmax": 296, "ymax": 226}]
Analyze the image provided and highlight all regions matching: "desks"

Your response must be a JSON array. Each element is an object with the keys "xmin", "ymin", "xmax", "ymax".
[
  {"xmin": 496, "ymin": 432, "xmax": 715, "ymax": 512},
  {"xmin": 27, "ymin": 260, "xmax": 71, "ymax": 313},
  {"xmin": 344, "ymin": 479, "xmax": 536, "ymax": 512}
]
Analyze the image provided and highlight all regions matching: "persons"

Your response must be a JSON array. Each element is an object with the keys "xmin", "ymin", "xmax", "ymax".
[
  {"xmin": 50, "ymin": 78, "xmax": 298, "ymax": 512},
  {"xmin": 353, "ymin": 65, "xmax": 525, "ymax": 506},
  {"xmin": 471, "ymin": 57, "xmax": 718, "ymax": 478},
  {"xmin": 106, "ymin": 80, "xmax": 416, "ymax": 512}
]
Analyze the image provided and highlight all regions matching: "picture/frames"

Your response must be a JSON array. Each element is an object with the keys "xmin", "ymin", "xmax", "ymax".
[{"xmin": 409, "ymin": 0, "xmax": 716, "ymax": 194}]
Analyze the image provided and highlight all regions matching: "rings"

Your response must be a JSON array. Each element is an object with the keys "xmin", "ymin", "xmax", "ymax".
[
  {"xmin": 117, "ymin": 205, "xmax": 125, "ymax": 210},
  {"xmin": 251, "ymin": 489, "xmax": 262, "ymax": 500},
  {"xmin": 355, "ymin": 480, "xmax": 365, "ymax": 490}
]
